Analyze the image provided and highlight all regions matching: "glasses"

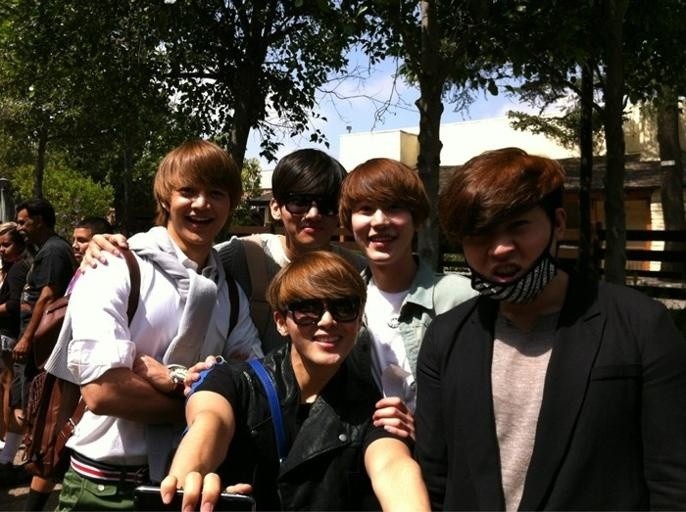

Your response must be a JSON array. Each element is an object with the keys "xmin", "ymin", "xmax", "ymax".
[
  {"xmin": 284, "ymin": 194, "xmax": 340, "ymax": 216},
  {"xmin": 287, "ymin": 294, "xmax": 364, "ymax": 327}
]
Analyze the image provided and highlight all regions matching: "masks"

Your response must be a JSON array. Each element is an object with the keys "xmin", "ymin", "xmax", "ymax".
[{"xmin": 463, "ymin": 213, "xmax": 560, "ymax": 305}]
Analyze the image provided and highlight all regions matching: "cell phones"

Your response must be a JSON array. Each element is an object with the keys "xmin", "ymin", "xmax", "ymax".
[{"xmin": 133, "ymin": 486, "xmax": 256, "ymax": 512}]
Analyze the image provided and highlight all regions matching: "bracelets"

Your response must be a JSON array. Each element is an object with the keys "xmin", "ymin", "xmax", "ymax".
[{"xmin": 173, "ymin": 377, "xmax": 184, "ymax": 397}]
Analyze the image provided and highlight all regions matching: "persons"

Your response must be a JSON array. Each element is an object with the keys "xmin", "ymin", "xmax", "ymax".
[
  {"xmin": 44, "ymin": 137, "xmax": 267, "ymax": 511},
  {"xmin": 1, "ymin": 221, "xmax": 33, "ymax": 470},
  {"xmin": 335, "ymin": 158, "xmax": 481, "ymax": 443},
  {"xmin": 160, "ymin": 249, "xmax": 431, "ymax": 512},
  {"xmin": 80, "ymin": 148, "xmax": 369, "ymax": 357},
  {"xmin": 0, "ymin": 197, "xmax": 79, "ymax": 482},
  {"xmin": 412, "ymin": 148, "xmax": 685, "ymax": 512},
  {"xmin": 23, "ymin": 214, "xmax": 115, "ymax": 510}
]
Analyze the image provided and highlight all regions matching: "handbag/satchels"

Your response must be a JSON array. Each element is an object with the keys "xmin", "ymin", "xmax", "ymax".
[{"xmin": 26, "ymin": 295, "xmax": 82, "ymax": 480}]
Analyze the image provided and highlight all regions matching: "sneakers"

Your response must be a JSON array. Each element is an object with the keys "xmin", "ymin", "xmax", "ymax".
[{"xmin": 0, "ymin": 461, "xmax": 31, "ymax": 488}]
